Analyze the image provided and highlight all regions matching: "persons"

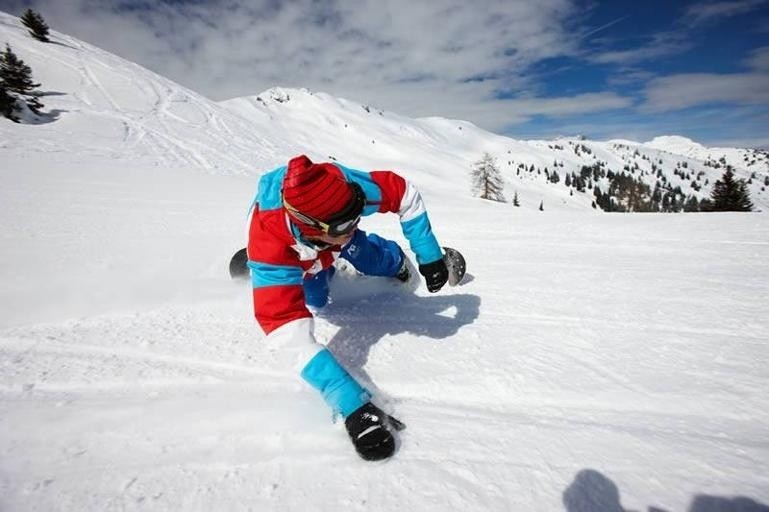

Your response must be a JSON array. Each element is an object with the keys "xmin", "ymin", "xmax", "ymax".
[{"xmin": 245, "ymin": 153, "xmax": 451, "ymax": 460}]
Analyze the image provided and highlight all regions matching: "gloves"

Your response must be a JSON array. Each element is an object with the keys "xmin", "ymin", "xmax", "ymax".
[
  {"xmin": 417, "ymin": 257, "xmax": 451, "ymax": 295},
  {"xmin": 343, "ymin": 399, "xmax": 409, "ymax": 463}
]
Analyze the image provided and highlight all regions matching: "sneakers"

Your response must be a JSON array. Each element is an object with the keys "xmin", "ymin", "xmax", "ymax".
[{"xmin": 395, "ymin": 260, "xmax": 413, "ymax": 283}]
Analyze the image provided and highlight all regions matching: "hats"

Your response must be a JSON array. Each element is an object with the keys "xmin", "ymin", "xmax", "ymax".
[{"xmin": 282, "ymin": 153, "xmax": 355, "ymax": 237}]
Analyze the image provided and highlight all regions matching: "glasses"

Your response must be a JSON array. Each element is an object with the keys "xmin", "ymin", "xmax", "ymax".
[{"xmin": 326, "ymin": 178, "xmax": 368, "ymax": 238}]
